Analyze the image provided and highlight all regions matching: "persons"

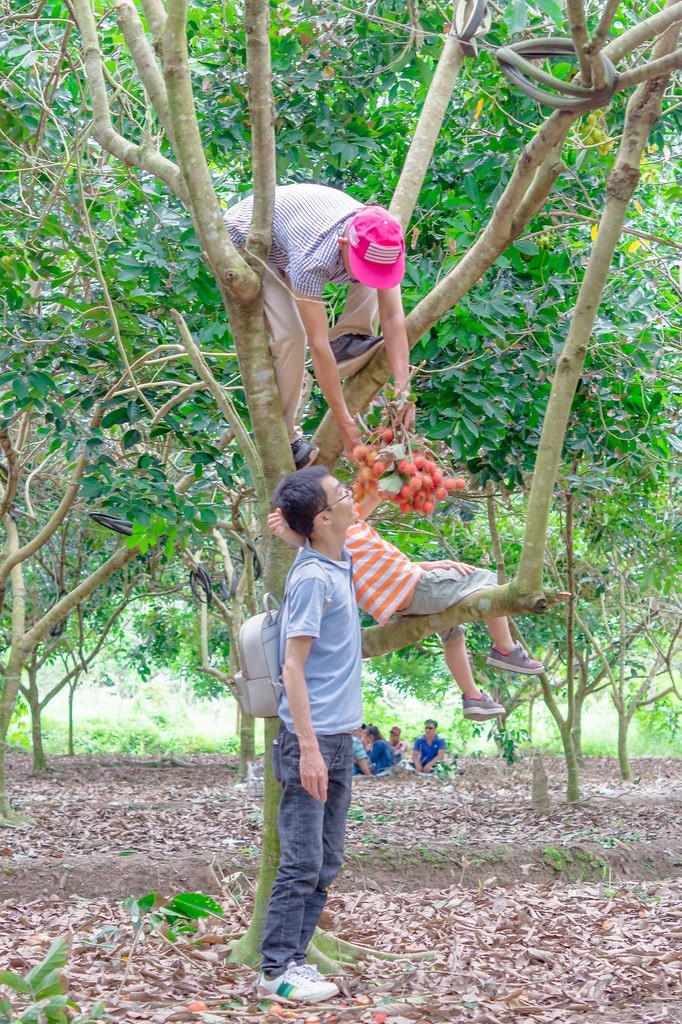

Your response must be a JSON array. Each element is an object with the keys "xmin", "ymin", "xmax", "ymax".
[
  {"xmin": 259, "ymin": 465, "xmax": 364, "ymax": 1005},
  {"xmin": 353, "ymin": 727, "xmax": 371, "ymax": 776},
  {"xmin": 397, "ymin": 720, "xmax": 445, "ymax": 776},
  {"xmin": 388, "ymin": 727, "xmax": 403, "ymax": 762},
  {"xmin": 223, "ymin": 183, "xmax": 416, "ymax": 468},
  {"xmin": 268, "ymin": 489, "xmax": 544, "ymax": 720},
  {"xmin": 365, "ymin": 726, "xmax": 393, "ymax": 775}
]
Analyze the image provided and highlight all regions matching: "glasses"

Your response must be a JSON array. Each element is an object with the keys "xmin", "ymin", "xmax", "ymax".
[
  {"xmin": 311, "ymin": 483, "xmax": 353, "ymax": 519},
  {"xmin": 425, "ymin": 726, "xmax": 435, "ymax": 730}
]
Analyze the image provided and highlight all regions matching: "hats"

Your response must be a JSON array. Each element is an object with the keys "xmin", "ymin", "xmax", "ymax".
[
  {"xmin": 347, "ymin": 207, "xmax": 406, "ymax": 290},
  {"xmin": 389, "ymin": 727, "xmax": 401, "ymax": 736}
]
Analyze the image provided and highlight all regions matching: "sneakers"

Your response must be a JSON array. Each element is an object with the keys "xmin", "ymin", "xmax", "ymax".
[
  {"xmin": 291, "ymin": 436, "xmax": 319, "ymax": 472},
  {"xmin": 330, "ymin": 331, "xmax": 385, "ymax": 378},
  {"xmin": 462, "ymin": 689, "xmax": 507, "ymax": 721},
  {"xmin": 256, "ymin": 959, "xmax": 340, "ymax": 1004},
  {"xmin": 486, "ymin": 639, "xmax": 544, "ymax": 674}
]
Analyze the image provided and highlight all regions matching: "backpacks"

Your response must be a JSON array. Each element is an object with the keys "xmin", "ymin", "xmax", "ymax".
[{"xmin": 234, "ymin": 562, "xmax": 331, "ymax": 717}]
